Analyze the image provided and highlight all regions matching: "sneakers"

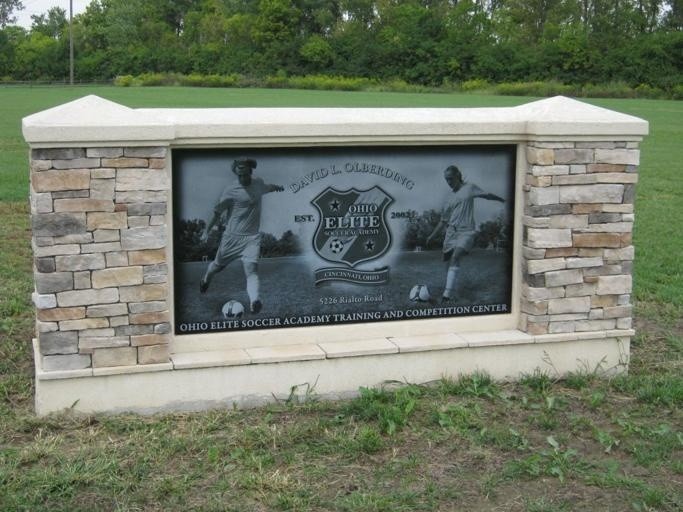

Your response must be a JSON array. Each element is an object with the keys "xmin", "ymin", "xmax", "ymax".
[
  {"xmin": 442, "ymin": 289, "xmax": 455, "ymax": 300},
  {"xmin": 250, "ymin": 299, "xmax": 263, "ymax": 315},
  {"xmin": 200, "ymin": 279, "xmax": 207, "ymax": 294}
]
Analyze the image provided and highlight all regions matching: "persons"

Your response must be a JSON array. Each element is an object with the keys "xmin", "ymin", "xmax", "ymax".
[
  {"xmin": 194, "ymin": 155, "xmax": 284, "ymax": 316},
  {"xmin": 422, "ymin": 163, "xmax": 503, "ymax": 303}
]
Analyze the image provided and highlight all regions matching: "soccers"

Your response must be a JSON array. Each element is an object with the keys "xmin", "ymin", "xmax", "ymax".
[
  {"xmin": 223, "ymin": 300, "xmax": 244, "ymax": 320},
  {"xmin": 409, "ymin": 285, "xmax": 430, "ymax": 303}
]
